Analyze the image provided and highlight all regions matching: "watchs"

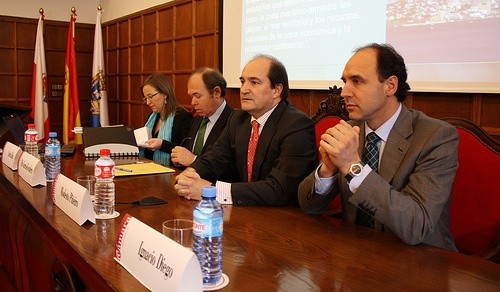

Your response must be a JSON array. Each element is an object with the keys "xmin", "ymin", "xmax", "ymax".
[{"xmin": 343, "ymin": 161, "xmax": 367, "ymax": 182}]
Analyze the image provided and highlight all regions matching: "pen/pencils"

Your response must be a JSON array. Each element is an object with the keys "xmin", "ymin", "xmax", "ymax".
[
  {"xmin": 115, "ymin": 167, "xmax": 133, "ymax": 172},
  {"xmin": 118, "ymin": 161, "xmax": 144, "ymax": 165}
]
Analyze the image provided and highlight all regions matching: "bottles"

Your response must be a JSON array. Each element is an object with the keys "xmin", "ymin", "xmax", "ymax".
[
  {"xmin": 45, "ymin": 132, "xmax": 60, "ymax": 180},
  {"xmin": 192, "ymin": 186, "xmax": 223, "ymax": 286},
  {"xmin": 95, "ymin": 149, "xmax": 116, "ymax": 215},
  {"xmin": 24, "ymin": 123, "xmax": 39, "ymax": 158}
]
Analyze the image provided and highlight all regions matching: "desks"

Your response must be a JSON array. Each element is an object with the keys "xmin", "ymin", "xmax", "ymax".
[{"xmin": 0, "ymin": 143, "xmax": 500, "ymax": 292}]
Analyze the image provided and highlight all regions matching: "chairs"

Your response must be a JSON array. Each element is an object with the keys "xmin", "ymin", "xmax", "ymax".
[
  {"xmin": 437, "ymin": 118, "xmax": 500, "ymax": 265},
  {"xmin": 310, "ymin": 85, "xmax": 351, "ymax": 219}
]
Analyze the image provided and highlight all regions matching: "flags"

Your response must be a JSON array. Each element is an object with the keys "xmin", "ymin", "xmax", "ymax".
[
  {"xmin": 90, "ymin": 10, "xmax": 111, "ymax": 127},
  {"xmin": 62, "ymin": 15, "xmax": 84, "ymax": 144},
  {"xmin": 29, "ymin": 15, "xmax": 50, "ymax": 144}
]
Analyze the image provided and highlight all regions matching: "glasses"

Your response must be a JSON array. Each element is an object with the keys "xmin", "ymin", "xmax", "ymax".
[{"xmin": 143, "ymin": 91, "xmax": 161, "ymax": 102}]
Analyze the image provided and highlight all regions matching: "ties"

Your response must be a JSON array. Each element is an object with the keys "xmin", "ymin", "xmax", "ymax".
[
  {"xmin": 192, "ymin": 116, "xmax": 210, "ymax": 157},
  {"xmin": 352, "ymin": 132, "xmax": 381, "ymax": 230},
  {"xmin": 246, "ymin": 120, "xmax": 260, "ymax": 183}
]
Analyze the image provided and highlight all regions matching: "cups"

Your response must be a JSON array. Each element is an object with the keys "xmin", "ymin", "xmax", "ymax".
[
  {"xmin": 77, "ymin": 175, "xmax": 97, "ymax": 206},
  {"xmin": 34, "ymin": 154, "xmax": 45, "ymax": 165},
  {"xmin": 19, "ymin": 143, "xmax": 24, "ymax": 151},
  {"xmin": 162, "ymin": 218, "xmax": 192, "ymax": 250}
]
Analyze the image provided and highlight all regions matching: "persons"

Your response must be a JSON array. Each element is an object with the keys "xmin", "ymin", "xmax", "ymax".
[
  {"xmin": 170, "ymin": 67, "xmax": 235, "ymax": 168},
  {"xmin": 138, "ymin": 72, "xmax": 192, "ymax": 166},
  {"xmin": 297, "ymin": 43, "xmax": 459, "ymax": 253},
  {"xmin": 174, "ymin": 55, "xmax": 319, "ymax": 206}
]
